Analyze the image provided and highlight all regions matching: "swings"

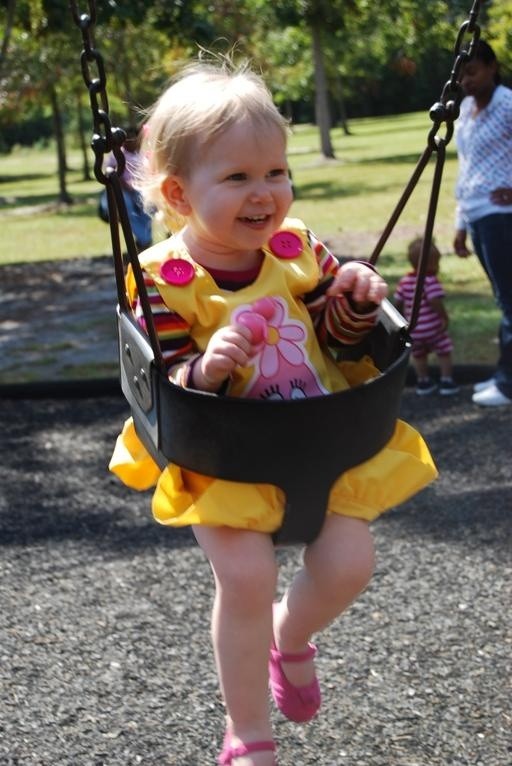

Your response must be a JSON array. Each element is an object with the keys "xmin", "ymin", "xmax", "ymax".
[{"xmin": 73, "ymin": 1, "xmax": 490, "ymax": 547}]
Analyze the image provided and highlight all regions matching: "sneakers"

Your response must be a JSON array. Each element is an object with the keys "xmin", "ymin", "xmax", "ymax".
[
  {"xmin": 471, "ymin": 378, "xmax": 511, "ymax": 406},
  {"xmin": 269, "ymin": 602, "xmax": 322, "ymax": 724},
  {"xmin": 216, "ymin": 730, "xmax": 280, "ymax": 766},
  {"xmin": 415, "ymin": 377, "xmax": 461, "ymax": 397}
]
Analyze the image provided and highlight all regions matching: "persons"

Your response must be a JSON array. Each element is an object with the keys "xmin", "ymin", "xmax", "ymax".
[
  {"xmin": 100, "ymin": 127, "xmax": 159, "ymax": 275},
  {"xmin": 390, "ymin": 236, "xmax": 459, "ymax": 397},
  {"xmin": 452, "ymin": 41, "xmax": 512, "ymax": 407},
  {"xmin": 105, "ymin": 46, "xmax": 442, "ymax": 766}
]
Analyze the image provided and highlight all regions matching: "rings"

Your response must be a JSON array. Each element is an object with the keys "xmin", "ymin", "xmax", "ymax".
[{"xmin": 501, "ymin": 194, "xmax": 508, "ymax": 200}]
